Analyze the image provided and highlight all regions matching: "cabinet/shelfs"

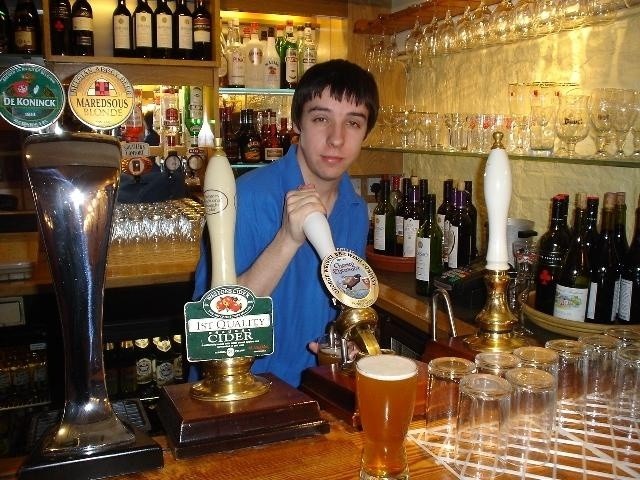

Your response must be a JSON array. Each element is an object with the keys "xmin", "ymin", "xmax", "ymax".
[
  {"xmin": 353, "ymin": 1, "xmax": 639, "ymax": 172},
  {"xmin": 0, "ymin": 1, "xmax": 348, "ymax": 219}
]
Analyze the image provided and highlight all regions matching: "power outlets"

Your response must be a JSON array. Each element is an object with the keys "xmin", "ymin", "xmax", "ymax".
[
  {"xmin": 367, "ymin": 202, "xmax": 379, "ymax": 221},
  {"xmin": 367, "ymin": 177, "xmax": 382, "ymax": 196}
]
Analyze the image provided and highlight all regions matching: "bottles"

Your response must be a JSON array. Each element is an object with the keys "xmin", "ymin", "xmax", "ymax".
[
  {"xmin": 510, "ymin": 192, "xmax": 640, "ymax": 327},
  {"xmin": 91, "ymin": 87, "xmax": 290, "ymax": 165},
  {"xmin": 0, "ymin": 0, "xmax": 213, "ymax": 61},
  {"xmin": 219, "ymin": 17, "xmax": 317, "ymax": 91},
  {"xmin": 372, "ymin": 174, "xmax": 478, "ymax": 296},
  {"xmin": 0, "ymin": 327, "xmax": 190, "ymax": 408}
]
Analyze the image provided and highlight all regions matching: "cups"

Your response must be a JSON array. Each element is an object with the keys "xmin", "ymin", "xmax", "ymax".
[
  {"xmin": 355, "ymin": 355, "xmax": 419, "ymax": 480},
  {"xmin": 422, "ymin": 326, "xmax": 640, "ymax": 480},
  {"xmin": 104, "ymin": 196, "xmax": 207, "ymax": 252}
]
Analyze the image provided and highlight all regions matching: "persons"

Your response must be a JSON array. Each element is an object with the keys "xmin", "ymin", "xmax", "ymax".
[{"xmin": 186, "ymin": 58, "xmax": 380, "ymax": 389}]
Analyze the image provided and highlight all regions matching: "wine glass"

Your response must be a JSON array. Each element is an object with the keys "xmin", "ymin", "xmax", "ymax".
[
  {"xmin": 361, "ymin": 81, "xmax": 640, "ymax": 162},
  {"xmin": 363, "ymin": 0, "xmax": 639, "ymax": 73}
]
